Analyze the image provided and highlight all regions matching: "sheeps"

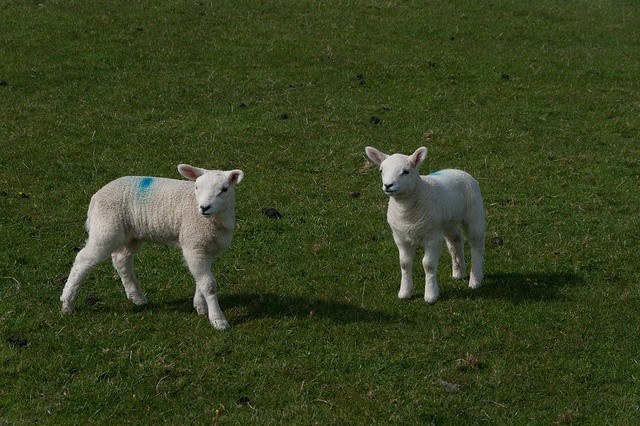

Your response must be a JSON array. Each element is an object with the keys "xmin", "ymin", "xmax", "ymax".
[
  {"xmin": 59, "ymin": 165, "xmax": 245, "ymax": 332},
  {"xmin": 364, "ymin": 146, "xmax": 486, "ymax": 305}
]
